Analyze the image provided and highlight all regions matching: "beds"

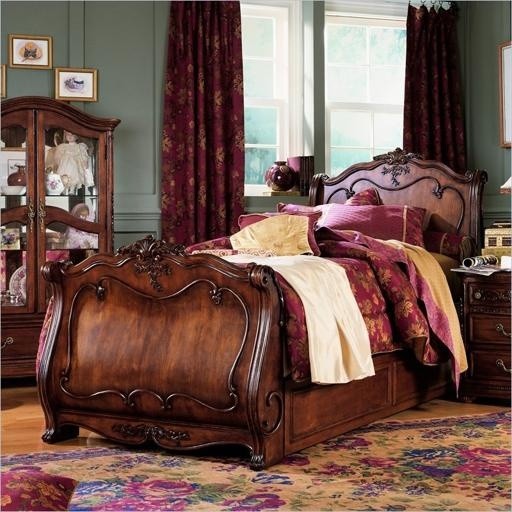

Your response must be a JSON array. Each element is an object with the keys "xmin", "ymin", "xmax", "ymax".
[{"xmin": 36, "ymin": 148, "xmax": 488, "ymax": 469}]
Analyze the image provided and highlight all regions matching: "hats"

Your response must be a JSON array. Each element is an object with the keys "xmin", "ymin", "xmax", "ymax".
[{"xmin": 19, "ymin": 42, "xmax": 43, "ymax": 60}]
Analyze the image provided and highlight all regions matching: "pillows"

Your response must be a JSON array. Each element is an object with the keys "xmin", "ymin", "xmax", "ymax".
[
  {"xmin": 0, "ymin": 469, "xmax": 80, "ymax": 511},
  {"xmin": 238, "ymin": 187, "xmax": 471, "ymax": 265}
]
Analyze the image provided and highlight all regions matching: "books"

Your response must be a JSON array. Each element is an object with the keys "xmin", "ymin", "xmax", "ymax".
[{"xmin": 449, "ymin": 252, "xmax": 511, "ymax": 277}]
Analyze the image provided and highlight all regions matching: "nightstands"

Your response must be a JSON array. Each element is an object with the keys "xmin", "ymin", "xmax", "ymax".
[{"xmin": 458, "ymin": 272, "xmax": 512, "ymax": 402}]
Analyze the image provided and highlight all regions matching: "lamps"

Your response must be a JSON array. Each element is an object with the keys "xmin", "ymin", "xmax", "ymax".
[{"xmin": 500, "ymin": 177, "xmax": 512, "ymax": 195}]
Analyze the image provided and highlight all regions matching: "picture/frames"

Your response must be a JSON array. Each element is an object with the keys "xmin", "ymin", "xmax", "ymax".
[
  {"xmin": 0, "ymin": 64, "xmax": 8, "ymax": 99},
  {"xmin": 1, "ymin": 147, "xmax": 27, "ymax": 196},
  {"xmin": 8, "ymin": 34, "xmax": 52, "ymax": 70},
  {"xmin": 1, "ymin": 228, "xmax": 21, "ymax": 251},
  {"xmin": 54, "ymin": 68, "xmax": 98, "ymax": 102},
  {"xmin": 498, "ymin": 41, "xmax": 512, "ymax": 148}
]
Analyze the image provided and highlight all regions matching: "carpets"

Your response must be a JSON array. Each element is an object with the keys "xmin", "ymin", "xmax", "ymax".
[{"xmin": 0, "ymin": 412, "xmax": 512, "ymax": 512}]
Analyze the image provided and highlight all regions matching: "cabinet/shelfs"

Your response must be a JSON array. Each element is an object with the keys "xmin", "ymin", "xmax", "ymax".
[{"xmin": 1, "ymin": 96, "xmax": 120, "ymax": 380}]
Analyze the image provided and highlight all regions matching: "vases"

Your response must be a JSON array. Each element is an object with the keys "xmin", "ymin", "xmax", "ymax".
[
  {"xmin": 264, "ymin": 161, "xmax": 296, "ymax": 191},
  {"xmin": 7, "ymin": 165, "xmax": 27, "ymax": 186}
]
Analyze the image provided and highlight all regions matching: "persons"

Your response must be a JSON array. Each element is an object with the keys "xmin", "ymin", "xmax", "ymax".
[
  {"xmin": 49, "ymin": 128, "xmax": 96, "ymax": 196},
  {"xmin": 62, "ymin": 202, "xmax": 98, "ymax": 249}
]
